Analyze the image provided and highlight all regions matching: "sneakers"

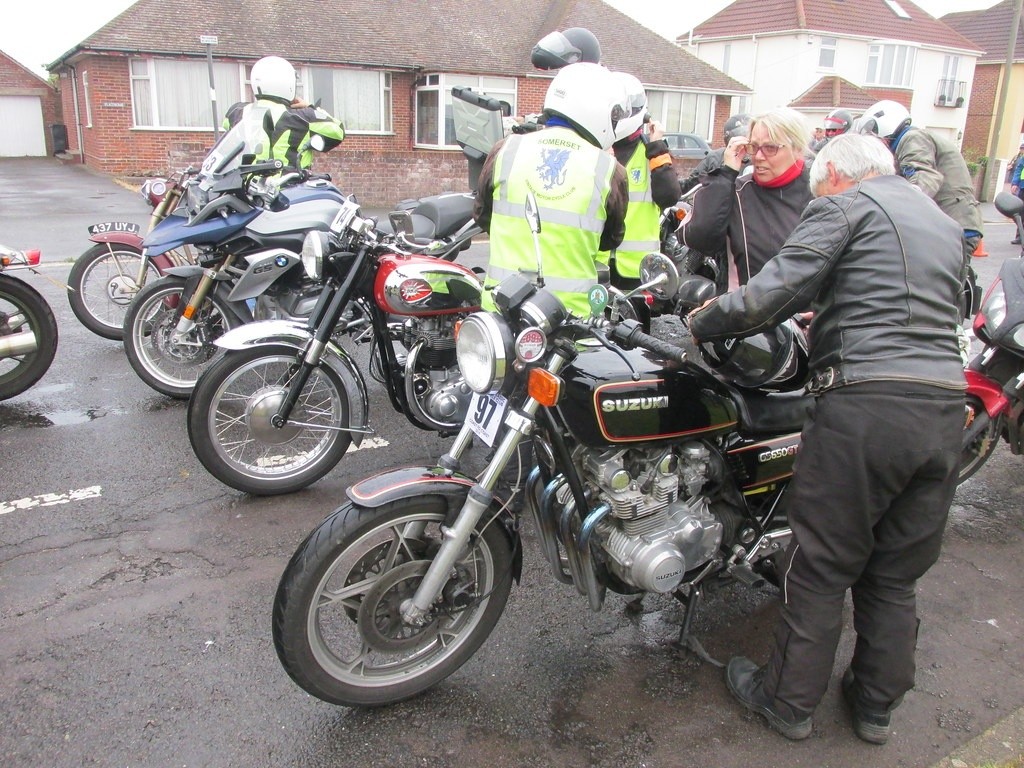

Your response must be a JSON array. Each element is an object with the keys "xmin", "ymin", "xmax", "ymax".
[
  {"xmin": 725, "ymin": 656, "xmax": 813, "ymax": 740},
  {"xmin": 843, "ymin": 666, "xmax": 893, "ymax": 743}
]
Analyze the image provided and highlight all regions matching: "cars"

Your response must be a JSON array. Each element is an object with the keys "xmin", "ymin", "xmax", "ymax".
[{"xmin": 662, "ymin": 132, "xmax": 710, "ymax": 157}]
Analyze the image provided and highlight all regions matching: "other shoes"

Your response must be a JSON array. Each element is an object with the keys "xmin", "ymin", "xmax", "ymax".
[{"xmin": 1011, "ymin": 237, "xmax": 1022, "ymax": 244}]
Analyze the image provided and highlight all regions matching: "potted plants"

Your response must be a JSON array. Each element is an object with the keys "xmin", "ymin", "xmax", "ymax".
[
  {"xmin": 955, "ymin": 97, "xmax": 964, "ymax": 107},
  {"xmin": 938, "ymin": 95, "xmax": 947, "ymax": 107}
]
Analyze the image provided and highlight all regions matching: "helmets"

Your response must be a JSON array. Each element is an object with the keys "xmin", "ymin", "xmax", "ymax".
[
  {"xmin": 542, "ymin": 62, "xmax": 616, "ymax": 150},
  {"xmin": 824, "ymin": 109, "xmax": 852, "ymax": 141},
  {"xmin": 531, "ymin": 27, "xmax": 601, "ymax": 69},
  {"xmin": 250, "ymin": 56, "xmax": 299, "ymax": 107},
  {"xmin": 858, "ymin": 100, "xmax": 911, "ymax": 138},
  {"xmin": 611, "ymin": 71, "xmax": 649, "ymax": 144},
  {"xmin": 723, "ymin": 112, "xmax": 754, "ymax": 146}
]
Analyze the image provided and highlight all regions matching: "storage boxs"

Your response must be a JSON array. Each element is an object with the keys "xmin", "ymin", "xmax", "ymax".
[{"xmin": 450, "ymin": 86, "xmax": 505, "ymax": 196}]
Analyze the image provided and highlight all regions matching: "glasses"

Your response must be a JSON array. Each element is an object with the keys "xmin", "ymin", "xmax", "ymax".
[
  {"xmin": 744, "ymin": 143, "xmax": 786, "ymax": 158},
  {"xmin": 827, "ymin": 129, "xmax": 837, "ymax": 132}
]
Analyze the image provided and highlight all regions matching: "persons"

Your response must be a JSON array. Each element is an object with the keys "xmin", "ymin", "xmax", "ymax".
[
  {"xmin": 480, "ymin": 60, "xmax": 984, "ymax": 745},
  {"xmin": 221, "ymin": 55, "xmax": 346, "ymax": 186},
  {"xmin": 1011, "ymin": 143, "xmax": 1024, "ymax": 244}
]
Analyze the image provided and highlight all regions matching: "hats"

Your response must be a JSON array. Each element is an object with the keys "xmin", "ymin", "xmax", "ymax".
[{"xmin": 1020, "ymin": 143, "xmax": 1024, "ymax": 148}]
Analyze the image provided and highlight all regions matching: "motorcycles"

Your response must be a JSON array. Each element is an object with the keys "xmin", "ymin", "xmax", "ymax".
[
  {"xmin": 272, "ymin": 194, "xmax": 815, "ymax": 707},
  {"xmin": 956, "ymin": 192, "xmax": 1024, "ymax": 486},
  {"xmin": 67, "ymin": 166, "xmax": 200, "ymax": 341},
  {"xmin": 188, "ymin": 194, "xmax": 693, "ymax": 497},
  {"xmin": 0, "ymin": 243, "xmax": 76, "ymax": 400},
  {"xmin": 123, "ymin": 86, "xmax": 503, "ymax": 398}
]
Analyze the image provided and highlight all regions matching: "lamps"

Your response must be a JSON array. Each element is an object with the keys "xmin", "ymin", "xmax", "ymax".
[{"xmin": 958, "ymin": 129, "xmax": 962, "ymax": 139}]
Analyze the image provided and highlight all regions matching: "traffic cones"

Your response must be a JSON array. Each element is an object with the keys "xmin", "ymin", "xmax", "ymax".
[{"xmin": 973, "ymin": 238, "xmax": 988, "ymax": 257}]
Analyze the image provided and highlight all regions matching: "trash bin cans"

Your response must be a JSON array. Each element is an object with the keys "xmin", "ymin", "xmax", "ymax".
[{"xmin": 49, "ymin": 125, "xmax": 66, "ymax": 156}]
